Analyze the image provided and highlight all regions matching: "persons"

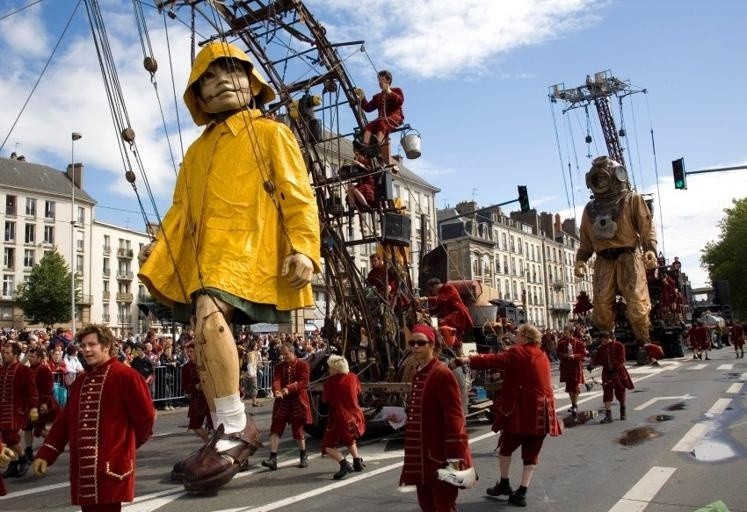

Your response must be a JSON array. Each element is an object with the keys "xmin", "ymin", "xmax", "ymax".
[
  {"xmin": 556, "ymin": 327, "xmax": 586, "ymax": 415},
  {"xmin": 0, "ymin": 321, "xmax": 213, "ymax": 512},
  {"xmin": 261, "ymin": 342, "xmax": 310, "ymax": 469},
  {"xmin": 368, "ymin": 253, "xmax": 409, "ymax": 316},
  {"xmin": 387, "ymin": 325, "xmax": 473, "ymax": 512},
  {"xmin": 353, "ymin": 70, "xmax": 405, "ymax": 152},
  {"xmin": 575, "ymin": 156, "xmax": 661, "ymax": 366},
  {"xmin": 419, "ymin": 277, "xmax": 474, "ymax": 374},
  {"xmin": 455, "ymin": 323, "xmax": 562, "ymax": 507},
  {"xmin": 484, "ymin": 309, "xmax": 520, "ymax": 424},
  {"xmin": 660, "ymin": 251, "xmax": 683, "ymax": 324},
  {"xmin": 345, "ymin": 147, "xmax": 378, "ymax": 216},
  {"xmin": 234, "ymin": 324, "xmax": 329, "ymax": 407},
  {"xmin": 640, "ymin": 340, "xmax": 664, "ymax": 366},
  {"xmin": 321, "ymin": 355, "xmax": 365, "ymax": 477},
  {"xmin": 139, "ymin": 38, "xmax": 321, "ymax": 494},
  {"xmin": 686, "ymin": 317, "xmax": 747, "ymax": 360},
  {"xmin": 588, "ymin": 331, "xmax": 634, "ymax": 423},
  {"xmin": 539, "ymin": 327, "xmax": 592, "ymax": 363}
]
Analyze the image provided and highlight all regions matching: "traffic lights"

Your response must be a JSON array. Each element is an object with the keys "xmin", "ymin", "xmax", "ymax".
[
  {"xmin": 517, "ymin": 182, "xmax": 530, "ymax": 212},
  {"xmin": 671, "ymin": 157, "xmax": 685, "ymax": 190}
]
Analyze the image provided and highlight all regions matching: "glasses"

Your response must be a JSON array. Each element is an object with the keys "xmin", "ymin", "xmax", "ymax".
[{"xmin": 408, "ymin": 340, "xmax": 430, "ymax": 346}]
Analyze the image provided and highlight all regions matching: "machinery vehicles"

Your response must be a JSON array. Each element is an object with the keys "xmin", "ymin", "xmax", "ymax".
[
  {"xmin": 80, "ymin": 1, "xmax": 526, "ymax": 434},
  {"xmin": 545, "ymin": 66, "xmax": 732, "ymax": 359}
]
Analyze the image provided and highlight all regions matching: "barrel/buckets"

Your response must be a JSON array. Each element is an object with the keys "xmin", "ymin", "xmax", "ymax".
[{"xmin": 400, "ymin": 127, "xmax": 422, "ymax": 159}]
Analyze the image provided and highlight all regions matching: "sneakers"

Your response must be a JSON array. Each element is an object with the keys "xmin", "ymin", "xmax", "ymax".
[
  {"xmin": 164, "ymin": 406, "xmax": 170, "ymax": 411},
  {"xmin": 509, "ymin": 491, "xmax": 527, "ymax": 506},
  {"xmin": 3, "ymin": 448, "xmax": 34, "ymax": 478},
  {"xmin": 170, "ymin": 406, "xmax": 174, "ymax": 410},
  {"xmin": 486, "ymin": 481, "xmax": 513, "ymax": 496},
  {"xmin": 353, "ymin": 139, "xmax": 365, "ymax": 148},
  {"xmin": 370, "ymin": 134, "xmax": 379, "ymax": 144},
  {"xmin": 568, "ymin": 406, "xmax": 578, "ymax": 415}
]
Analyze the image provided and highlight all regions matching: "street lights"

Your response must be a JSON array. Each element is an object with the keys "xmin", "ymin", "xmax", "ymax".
[{"xmin": 69, "ymin": 128, "xmax": 83, "ymax": 342}]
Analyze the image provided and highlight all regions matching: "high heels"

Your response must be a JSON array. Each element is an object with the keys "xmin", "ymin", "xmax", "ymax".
[
  {"xmin": 334, "ymin": 463, "xmax": 353, "ymax": 480},
  {"xmin": 353, "ymin": 461, "xmax": 366, "ymax": 472}
]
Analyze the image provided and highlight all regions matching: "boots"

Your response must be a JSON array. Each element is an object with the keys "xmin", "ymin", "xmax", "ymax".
[
  {"xmin": 600, "ymin": 410, "xmax": 613, "ymax": 425},
  {"xmin": 619, "ymin": 406, "xmax": 628, "ymax": 420}
]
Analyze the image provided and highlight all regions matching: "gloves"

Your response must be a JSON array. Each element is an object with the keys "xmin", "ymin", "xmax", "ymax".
[
  {"xmin": 29, "ymin": 408, "xmax": 39, "ymax": 422},
  {"xmin": 32, "ymin": 458, "xmax": 47, "ymax": 477},
  {"xmin": 1, "ymin": 447, "xmax": 15, "ymax": 469}
]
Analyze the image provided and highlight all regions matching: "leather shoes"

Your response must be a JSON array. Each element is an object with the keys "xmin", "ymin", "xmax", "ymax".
[
  {"xmin": 262, "ymin": 458, "xmax": 277, "ymax": 470},
  {"xmin": 301, "ymin": 453, "xmax": 308, "ymax": 468}
]
